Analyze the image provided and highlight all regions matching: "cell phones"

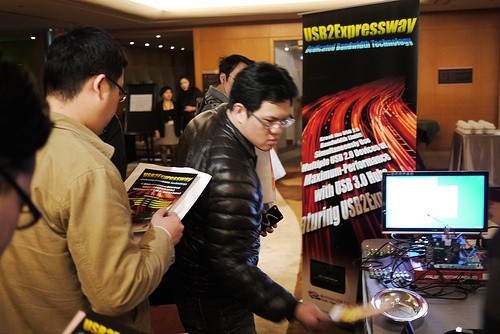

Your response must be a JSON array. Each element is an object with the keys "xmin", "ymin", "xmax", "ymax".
[{"xmin": 261, "ymin": 205, "xmax": 283, "ymax": 231}]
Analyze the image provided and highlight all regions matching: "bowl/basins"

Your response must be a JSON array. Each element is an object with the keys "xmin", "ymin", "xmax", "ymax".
[{"xmin": 371, "ymin": 287, "xmax": 429, "ymax": 322}]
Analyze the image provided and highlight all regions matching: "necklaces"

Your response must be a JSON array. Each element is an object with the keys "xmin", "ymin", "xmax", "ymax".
[{"xmin": 164, "ymin": 102, "xmax": 171, "ymax": 120}]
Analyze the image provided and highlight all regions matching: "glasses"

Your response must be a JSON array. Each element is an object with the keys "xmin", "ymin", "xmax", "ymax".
[
  {"xmin": 7, "ymin": 176, "xmax": 42, "ymax": 230},
  {"xmin": 248, "ymin": 110, "xmax": 296, "ymax": 131},
  {"xmin": 105, "ymin": 76, "xmax": 128, "ymax": 102}
]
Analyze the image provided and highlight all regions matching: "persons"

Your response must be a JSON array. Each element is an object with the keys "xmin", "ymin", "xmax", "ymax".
[
  {"xmin": 99, "ymin": 113, "xmax": 126, "ymax": 183},
  {"xmin": 0, "ymin": 28, "xmax": 184, "ymax": 334},
  {"xmin": 0, "ymin": 57, "xmax": 56, "ymax": 258},
  {"xmin": 176, "ymin": 76, "xmax": 204, "ymax": 135},
  {"xmin": 151, "ymin": 62, "xmax": 331, "ymax": 334},
  {"xmin": 192, "ymin": 54, "xmax": 273, "ymax": 211},
  {"xmin": 150, "ymin": 87, "xmax": 182, "ymax": 167}
]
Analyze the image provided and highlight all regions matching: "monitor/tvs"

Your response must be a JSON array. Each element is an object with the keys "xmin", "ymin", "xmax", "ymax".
[{"xmin": 382, "ymin": 172, "xmax": 489, "ymax": 237}]
